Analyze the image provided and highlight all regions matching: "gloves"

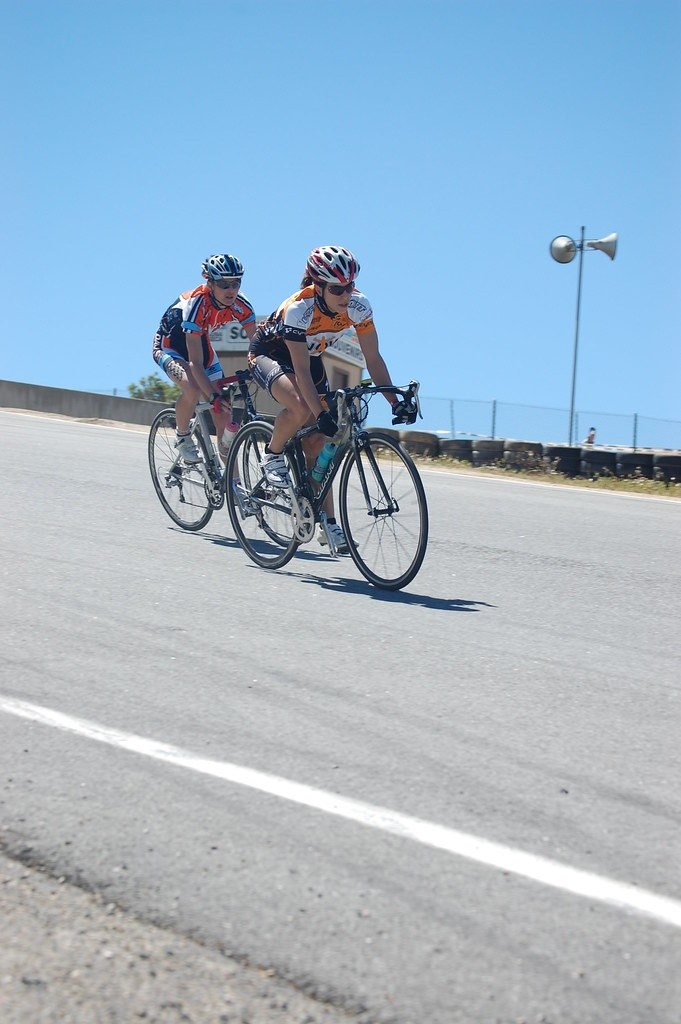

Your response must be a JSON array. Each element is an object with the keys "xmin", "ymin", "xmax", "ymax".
[
  {"xmin": 316, "ymin": 411, "xmax": 338, "ymax": 438},
  {"xmin": 391, "ymin": 402, "xmax": 417, "ymax": 425}
]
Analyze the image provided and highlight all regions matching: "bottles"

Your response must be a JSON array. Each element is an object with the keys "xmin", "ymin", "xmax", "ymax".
[
  {"xmin": 219, "ymin": 422, "xmax": 240, "ymax": 457},
  {"xmin": 311, "ymin": 442, "xmax": 337, "ymax": 482}
]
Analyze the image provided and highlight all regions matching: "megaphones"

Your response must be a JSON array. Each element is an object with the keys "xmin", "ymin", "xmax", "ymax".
[
  {"xmin": 587, "ymin": 231, "xmax": 619, "ymax": 261},
  {"xmin": 550, "ymin": 235, "xmax": 576, "ymax": 263}
]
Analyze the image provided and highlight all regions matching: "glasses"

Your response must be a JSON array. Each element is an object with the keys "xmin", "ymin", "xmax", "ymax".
[
  {"xmin": 213, "ymin": 280, "xmax": 241, "ymax": 290},
  {"xmin": 325, "ymin": 281, "xmax": 355, "ymax": 296}
]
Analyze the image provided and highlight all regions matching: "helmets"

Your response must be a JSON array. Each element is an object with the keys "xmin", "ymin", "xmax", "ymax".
[
  {"xmin": 305, "ymin": 246, "xmax": 360, "ymax": 284},
  {"xmin": 202, "ymin": 254, "xmax": 244, "ymax": 280}
]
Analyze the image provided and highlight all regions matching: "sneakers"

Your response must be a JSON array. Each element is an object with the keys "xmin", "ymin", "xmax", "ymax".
[
  {"xmin": 259, "ymin": 443, "xmax": 293, "ymax": 488},
  {"xmin": 174, "ymin": 437, "xmax": 204, "ymax": 462},
  {"xmin": 317, "ymin": 524, "xmax": 359, "ymax": 548},
  {"xmin": 222, "ymin": 477, "xmax": 245, "ymax": 504}
]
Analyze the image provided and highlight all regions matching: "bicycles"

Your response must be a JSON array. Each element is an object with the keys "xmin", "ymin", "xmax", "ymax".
[
  {"xmin": 148, "ymin": 368, "xmax": 302, "ymax": 547},
  {"xmin": 225, "ymin": 380, "xmax": 429, "ymax": 592}
]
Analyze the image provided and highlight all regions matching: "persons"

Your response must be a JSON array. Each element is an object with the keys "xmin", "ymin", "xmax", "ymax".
[
  {"xmin": 152, "ymin": 254, "xmax": 257, "ymax": 506},
  {"xmin": 587, "ymin": 427, "xmax": 596, "ymax": 444},
  {"xmin": 247, "ymin": 245, "xmax": 418, "ymax": 547}
]
